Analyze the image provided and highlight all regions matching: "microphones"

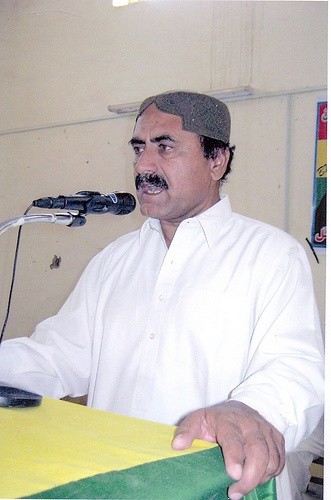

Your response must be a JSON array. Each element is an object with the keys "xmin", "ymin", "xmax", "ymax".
[{"xmin": 31, "ymin": 192, "xmax": 136, "ymax": 216}]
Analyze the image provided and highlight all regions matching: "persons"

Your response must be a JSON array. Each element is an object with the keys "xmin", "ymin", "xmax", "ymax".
[{"xmin": 0, "ymin": 91, "xmax": 326, "ymax": 500}]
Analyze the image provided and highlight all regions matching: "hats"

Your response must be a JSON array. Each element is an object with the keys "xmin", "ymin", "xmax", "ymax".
[{"xmin": 136, "ymin": 91, "xmax": 232, "ymax": 145}]
